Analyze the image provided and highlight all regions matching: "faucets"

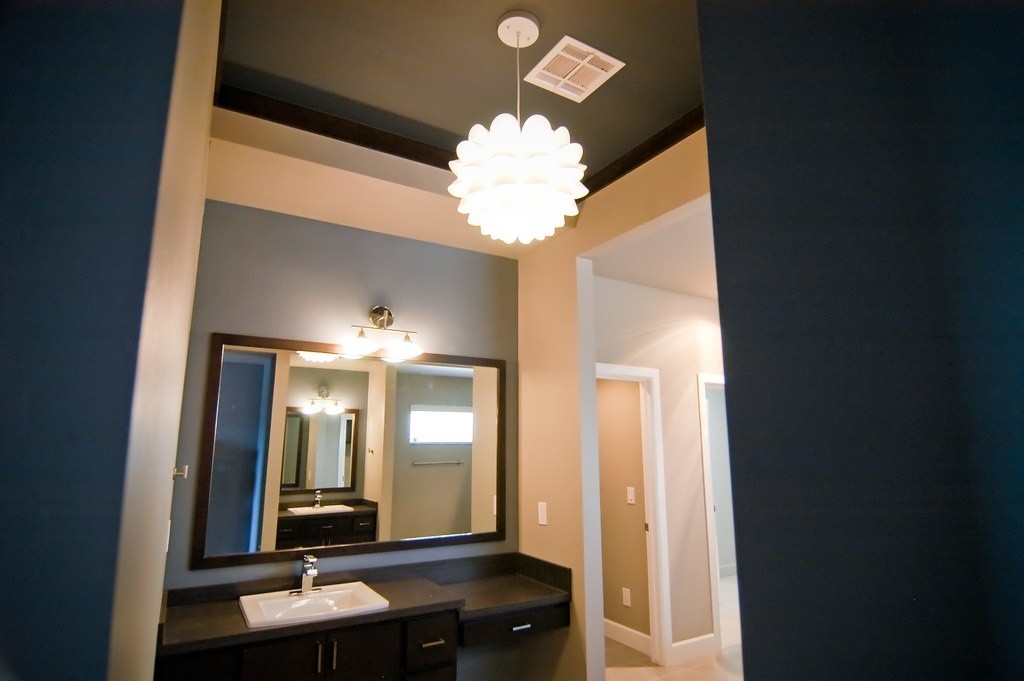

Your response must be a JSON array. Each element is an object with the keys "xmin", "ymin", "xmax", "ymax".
[
  {"xmin": 314, "ymin": 490, "xmax": 323, "ymax": 508},
  {"xmin": 301, "ymin": 554, "xmax": 319, "ymax": 595}
]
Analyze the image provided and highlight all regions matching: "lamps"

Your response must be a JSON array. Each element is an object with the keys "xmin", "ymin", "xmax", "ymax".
[
  {"xmin": 301, "ymin": 386, "xmax": 345, "ymax": 415},
  {"xmin": 448, "ymin": 9, "xmax": 591, "ymax": 243},
  {"xmin": 342, "ymin": 304, "xmax": 424, "ymax": 359},
  {"xmin": 297, "ymin": 349, "xmax": 339, "ymax": 363}
]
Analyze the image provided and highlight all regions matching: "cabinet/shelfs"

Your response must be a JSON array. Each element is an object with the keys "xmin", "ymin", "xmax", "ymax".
[
  {"xmin": 274, "ymin": 514, "xmax": 375, "ymax": 551},
  {"xmin": 154, "ymin": 601, "xmax": 571, "ymax": 680}
]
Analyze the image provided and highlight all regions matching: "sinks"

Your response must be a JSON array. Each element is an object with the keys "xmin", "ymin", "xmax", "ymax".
[
  {"xmin": 286, "ymin": 504, "xmax": 355, "ymax": 516},
  {"xmin": 237, "ymin": 580, "xmax": 391, "ymax": 629}
]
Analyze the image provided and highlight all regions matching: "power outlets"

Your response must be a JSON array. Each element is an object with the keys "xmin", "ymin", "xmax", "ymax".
[
  {"xmin": 627, "ymin": 487, "xmax": 635, "ymax": 504},
  {"xmin": 538, "ymin": 502, "xmax": 547, "ymax": 525},
  {"xmin": 623, "ymin": 588, "xmax": 631, "ymax": 607},
  {"xmin": 493, "ymin": 495, "xmax": 496, "ymax": 515}
]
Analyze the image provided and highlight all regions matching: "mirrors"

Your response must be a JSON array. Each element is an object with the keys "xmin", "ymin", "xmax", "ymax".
[
  {"xmin": 280, "ymin": 406, "xmax": 359, "ymax": 492},
  {"xmin": 188, "ymin": 333, "xmax": 505, "ymax": 570}
]
